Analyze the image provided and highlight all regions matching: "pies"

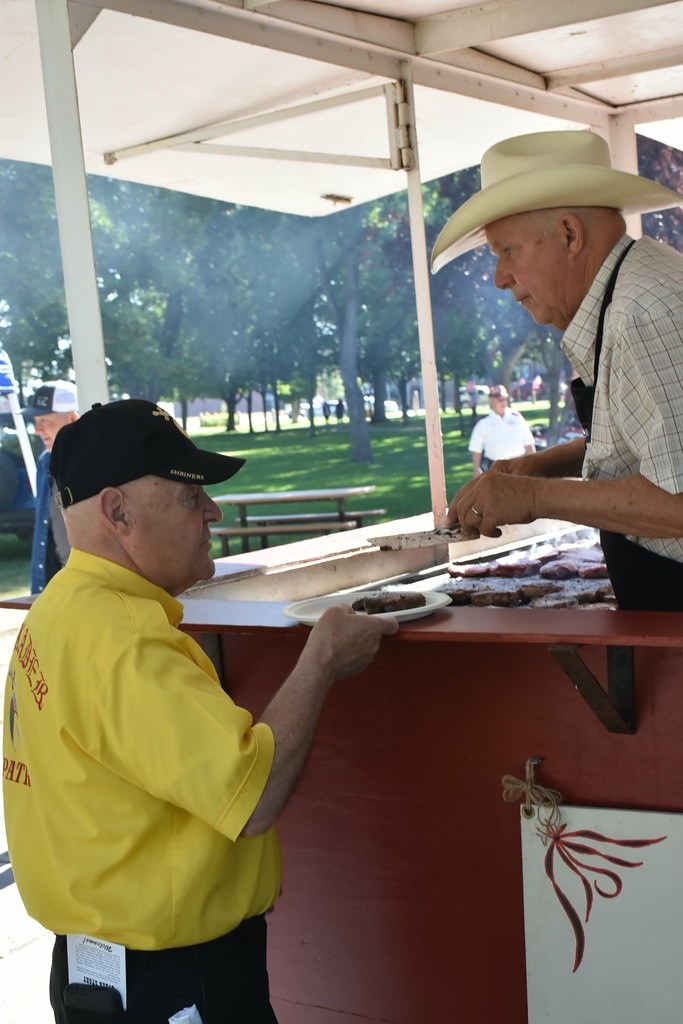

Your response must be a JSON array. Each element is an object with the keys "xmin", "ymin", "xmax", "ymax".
[{"xmin": 348, "ymin": 576, "xmax": 610, "ymax": 614}]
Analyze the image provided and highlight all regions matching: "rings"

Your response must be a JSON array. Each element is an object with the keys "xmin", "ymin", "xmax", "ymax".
[{"xmin": 472, "ymin": 503, "xmax": 482, "ymax": 518}]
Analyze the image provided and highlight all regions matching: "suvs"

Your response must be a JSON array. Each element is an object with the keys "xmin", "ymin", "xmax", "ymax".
[
  {"xmin": 459, "ymin": 385, "xmax": 492, "ymax": 409},
  {"xmin": 0, "ymin": 413, "xmax": 44, "ymax": 542}
]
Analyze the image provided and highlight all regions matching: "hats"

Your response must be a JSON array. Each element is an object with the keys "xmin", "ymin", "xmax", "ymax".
[
  {"xmin": 49, "ymin": 399, "xmax": 246, "ymax": 508},
  {"xmin": 488, "ymin": 384, "xmax": 508, "ymax": 397},
  {"xmin": 430, "ymin": 130, "xmax": 683, "ymax": 274},
  {"xmin": 15, "ymin": 379, "xmax": 79, "ymax": 415}
]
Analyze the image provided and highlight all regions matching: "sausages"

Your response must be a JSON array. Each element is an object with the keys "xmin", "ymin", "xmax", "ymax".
[{"xmin": 447, "ymin": 545, "xmax": 608, "ymax": 578}]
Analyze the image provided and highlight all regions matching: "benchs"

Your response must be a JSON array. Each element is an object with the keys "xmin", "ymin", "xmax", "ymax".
[
  {"xmin": 234, "ymin": 510, "xmax": 387, "ymax": 549},
  {"xmin": 209, "ymin": 521, "xmax": 357, "ymax": 557}
]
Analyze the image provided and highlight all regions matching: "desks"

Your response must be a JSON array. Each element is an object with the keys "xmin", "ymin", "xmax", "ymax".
[{"xmin": 210, "ymin": 485, "xmax": 378, "ymax": 553}]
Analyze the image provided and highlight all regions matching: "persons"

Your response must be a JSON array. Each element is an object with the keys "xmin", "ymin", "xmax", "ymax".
[
  {"xmin": 2, "ymin": 399, "xmax": 401, "ymax": 1024},
  {"xmin": 14, "ymin": 379, "xmax": 82, "ymax": 598},
  {"xmin": 322, "ymin": 396, "xmax": 348, "ymax": 428},
  {"xmin": 468, "ymin": 383, "xmax": 537, "ymax": 484},
  {"xmin": 430, "ymin": 132, "xmax": 683, "ymax": 610}
]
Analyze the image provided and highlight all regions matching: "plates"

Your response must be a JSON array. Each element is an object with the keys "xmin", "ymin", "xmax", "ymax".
[{"xmin": 283, "ymin": 590, "xmax": 453, "ymax": 627}]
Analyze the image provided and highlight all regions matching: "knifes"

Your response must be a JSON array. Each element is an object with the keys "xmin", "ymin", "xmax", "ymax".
[{"xmin": 367, "ymin": 525, "xmax": 482, "ymax": 549}]
